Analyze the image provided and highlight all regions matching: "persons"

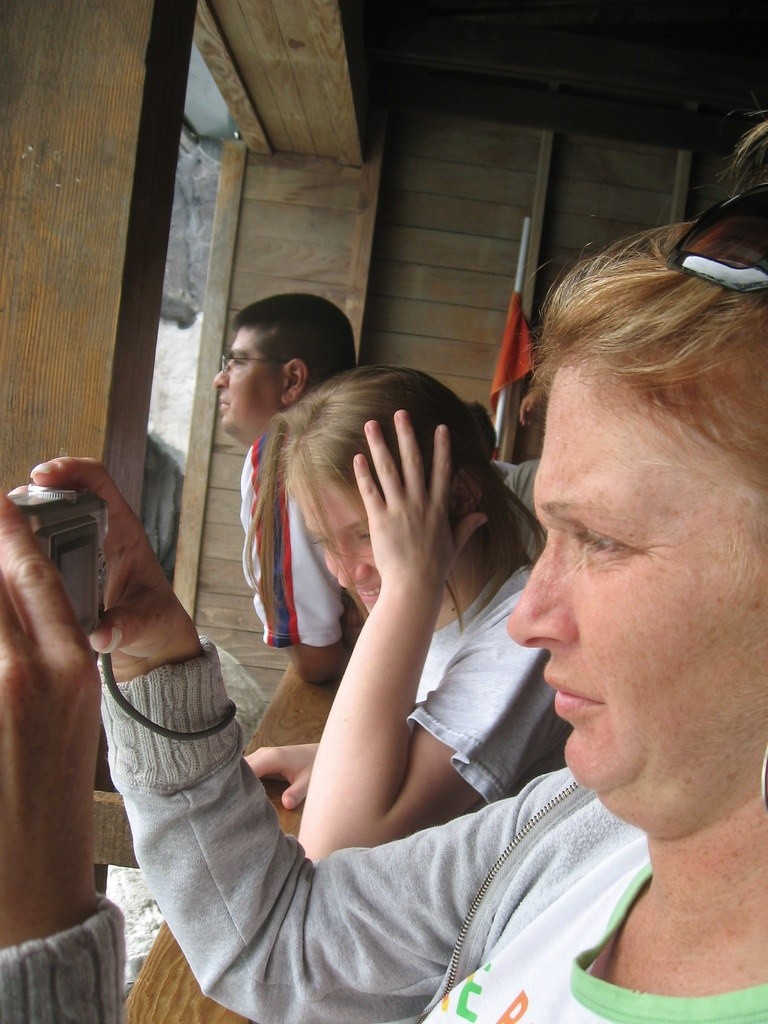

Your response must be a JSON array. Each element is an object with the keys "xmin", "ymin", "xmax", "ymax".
[
  {"xmin": 0, "ymin": 115, "xmax": 768, "ymax": 1024},
  {"xmin": 214, "ymin": 292, "xmax": 575, "ymax": 866}
]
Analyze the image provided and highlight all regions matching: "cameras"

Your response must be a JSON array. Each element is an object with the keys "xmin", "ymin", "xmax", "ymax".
[{"xmin": 7, "ymin": 484, "xmax": 108, "ymax": 639}]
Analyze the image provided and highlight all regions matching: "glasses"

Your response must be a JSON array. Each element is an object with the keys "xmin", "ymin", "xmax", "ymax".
[
  {"xmin": 666, "ymin": 183, "xmax": 768, "ymax": 293},
  {"xmin": 222, "ymin": 354, "xmax": 288, "ymax": 374}
]
